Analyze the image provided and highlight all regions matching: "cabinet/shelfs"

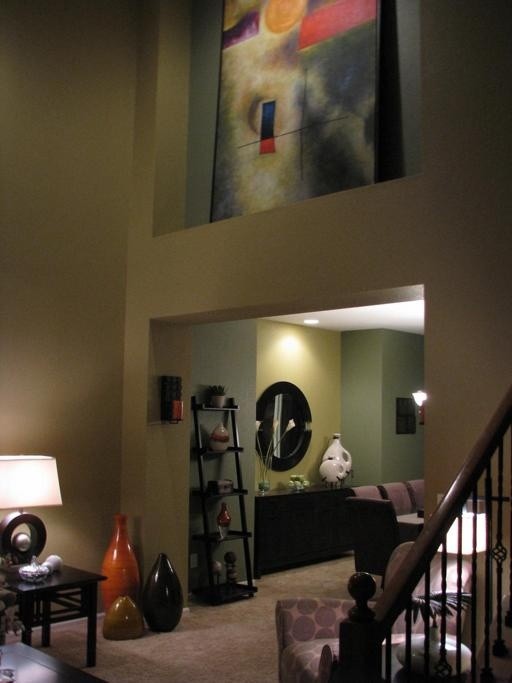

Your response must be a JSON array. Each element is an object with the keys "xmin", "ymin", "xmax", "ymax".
[
  {"xmin": 188, "ymin": 390, "xmax": 259, "ymax": 605},
  {"xmin": 252, "ymin": 482, "xmax": 361, "ymax": 580}
]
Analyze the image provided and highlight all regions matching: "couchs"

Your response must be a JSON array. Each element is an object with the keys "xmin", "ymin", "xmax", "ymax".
[{"xmin": 343, "ymin": 478, "xmax": 427, "ymax": 577}]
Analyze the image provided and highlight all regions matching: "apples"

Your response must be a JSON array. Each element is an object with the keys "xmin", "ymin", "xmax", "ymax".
[{"xmin": 288, "ymin": 475, "xmax": 309, "ymax": 486}]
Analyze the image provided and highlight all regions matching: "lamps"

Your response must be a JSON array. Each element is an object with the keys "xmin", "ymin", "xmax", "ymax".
[{"xmin": 0, "ymin": 454, "xmax": 65, "ymax": 567}]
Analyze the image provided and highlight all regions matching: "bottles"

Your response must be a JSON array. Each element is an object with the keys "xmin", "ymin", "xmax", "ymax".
[
  {"xmin": 216, "ymin": 502, "xmax": 232, "ymax": 540},
  {"xmin": 18, "ymin": 555, "xmax": 51, "ymax": 584},
  {"xmin": 208, "ymin": 421, "xmax": 231, "ymax": 452},
  {"xmin": 101, "ymin": 510, "xmax": 184, "ymax": 640},
  {"xmin": 318, "ymin": 455, "xmax": 347, "ymax": 483},
  {"xmin": 322, "ymin": 432, "xmax": 353, "ymax": 481}
]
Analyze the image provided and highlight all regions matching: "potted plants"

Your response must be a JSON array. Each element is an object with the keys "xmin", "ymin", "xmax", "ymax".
[{"xmin": 206, "ymin": 385, "xmax": 226, "ymax": 408}]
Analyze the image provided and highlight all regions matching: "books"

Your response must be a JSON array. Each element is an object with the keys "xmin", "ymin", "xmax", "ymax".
[
  {"xmin": 160, "ymin": 373, "xmax": 171, "ymax": 421},
  {"xmin": 171, "ymin": 375, "xmax": 176, "ymax": 419},
  {"xmin": 176, "ymin": 375, "xmax": 183, "ymax": 421}
]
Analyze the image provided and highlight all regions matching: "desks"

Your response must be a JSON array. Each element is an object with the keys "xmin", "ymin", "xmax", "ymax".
[{"xmin": 1, "ymin": 561, "xmax": 110, "ymax": 683}]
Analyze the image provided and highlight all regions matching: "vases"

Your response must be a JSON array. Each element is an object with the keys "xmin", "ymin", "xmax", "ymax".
[
  {"xmin": 100, "ymin": 511, "xmax": 184, "ymax": 642},
  {"xmin": 258, "ymin": 454, "xmax": 273, "ymax": 495},
  {"xmin": 394, "ymin": 625, "xmax": 472, "ymax": 679}
]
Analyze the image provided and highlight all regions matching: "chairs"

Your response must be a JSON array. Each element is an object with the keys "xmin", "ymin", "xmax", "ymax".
[{"xmin": 274, "ymin": 539, "xmax": 474, "ymax": 682}]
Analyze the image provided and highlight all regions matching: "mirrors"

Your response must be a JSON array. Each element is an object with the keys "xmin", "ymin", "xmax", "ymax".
[{"xmin": 255, "ymin": 381, "xmax": 314, "ymax": 473}]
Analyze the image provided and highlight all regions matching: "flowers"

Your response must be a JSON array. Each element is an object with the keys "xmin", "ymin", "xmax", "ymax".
[{"xmin": 253, "ymin": 418, "xmax": 296, "ymax": 481}]
